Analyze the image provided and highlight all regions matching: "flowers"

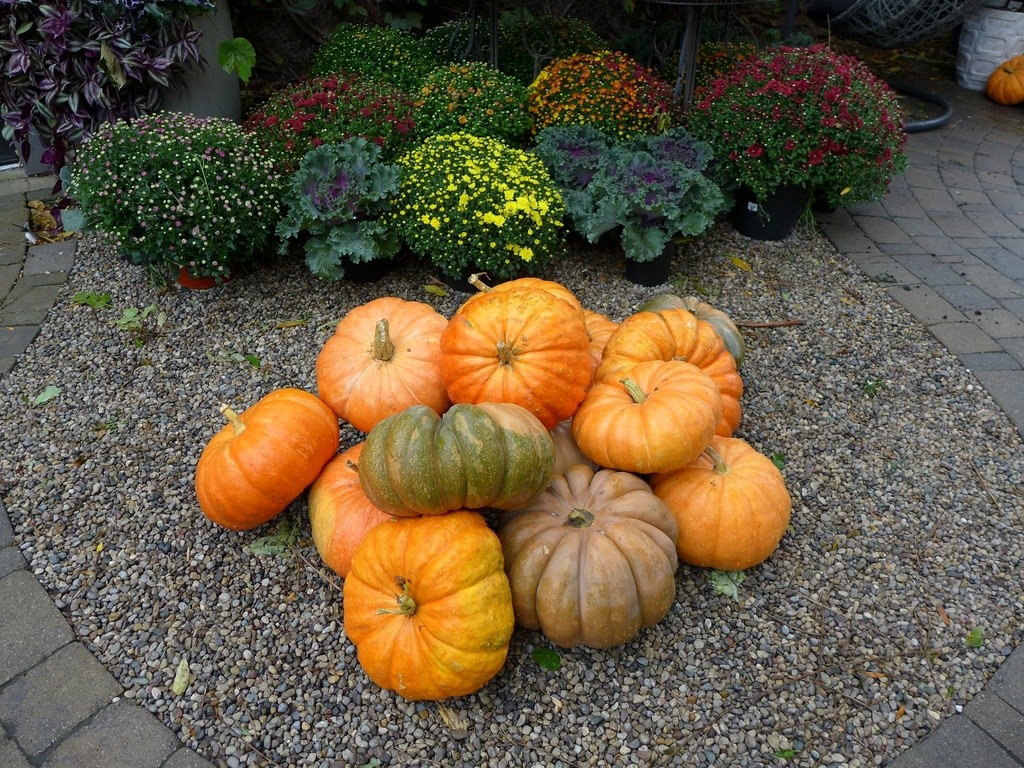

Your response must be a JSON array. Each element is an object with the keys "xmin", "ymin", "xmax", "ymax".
[{"xmin": 65, "ymin": 15, "xmax": 909, "ymax": 275}]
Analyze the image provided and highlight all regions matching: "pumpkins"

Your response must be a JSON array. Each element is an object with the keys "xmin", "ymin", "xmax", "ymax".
[
  {"xmin": 986, "ymin": 52, "xmax": 1024, "ymax": 106},
  {"xmin": 194, "ymin": 274, "xmax": 791, "ymax": 700}
]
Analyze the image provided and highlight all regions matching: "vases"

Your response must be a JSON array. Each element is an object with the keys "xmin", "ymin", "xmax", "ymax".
[
  {"xmin": 810, "ymin": 183, "xmax": 843, "ymax": 216},
  {"xmin": 8, "ymin": 120, "xmax": 67, "ymax": 176},
  {"xmin": 624, "ymin": 236, "xmax": 677, "ymax": 289},
  {"xmin": 954, "ymin": 0, "xmax": 1024, "ymax": 92},
  {"xmin": 170, "ymin": 255, "xmax": 239, "ymax": 290},
  {"xmin": 339, "ymin": 253, "xmax": 384, "ymax": 284},
  {"xmin": 433, "ymin": 254, "xmax": 501, "ymax": 292},
  {"xmin": 720, "ymin": 169, "xmax": 811, "ymax": 240}
]
